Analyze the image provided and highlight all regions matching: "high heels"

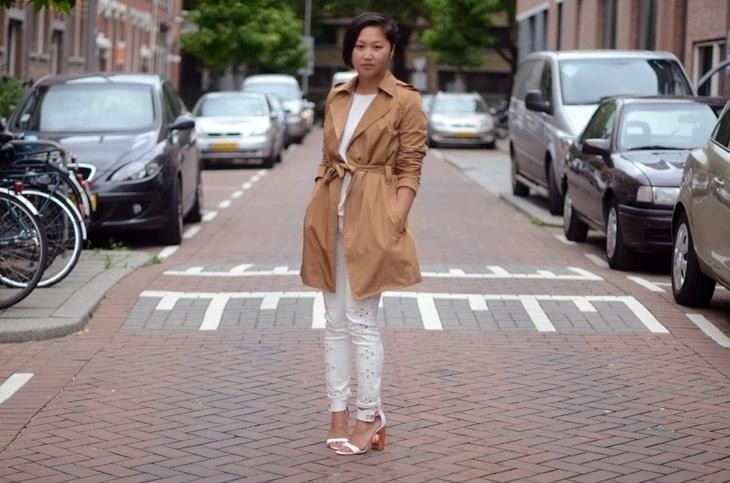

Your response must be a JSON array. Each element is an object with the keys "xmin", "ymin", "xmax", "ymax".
[
  {"xmin": 327, "ymin": 409, "xmax": 347, "ymax": 450},
  {"xmin": 336, "ymin": 411, "xmax": 386, "ymax": 456}
]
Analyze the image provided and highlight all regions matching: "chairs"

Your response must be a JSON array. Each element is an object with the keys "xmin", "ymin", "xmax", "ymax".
[{"xmin": 623, "ymin": 120, "xmax": 711, "ymax": 145}]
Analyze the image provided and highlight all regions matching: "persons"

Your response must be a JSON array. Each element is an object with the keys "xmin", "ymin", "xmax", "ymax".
[{"xmin": 297, "ymin": 11, "xmax": 430, "ymax": 459}]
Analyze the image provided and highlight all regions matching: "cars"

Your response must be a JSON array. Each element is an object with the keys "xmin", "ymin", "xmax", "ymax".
[
  {"xmin": 1, "ymin": 71, "xmax": 204, "ymax": 247},
  {"xmin": 192, "ymin": 73, "xmax": 316, "ymax": 169},
  {"xmin": 418, "ymin": 91, "xmax": 499, "ymax": 148},
  {"xmin": 558, "ymin": 93, "xmax": 719, "ymax": 269},
  {"xmin": 669, "ymin": 98, "xmax": 730, "ymax": 310}
]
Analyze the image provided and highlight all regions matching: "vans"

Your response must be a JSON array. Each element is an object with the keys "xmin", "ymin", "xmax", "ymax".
[{"xmin": 507, "ymin": 48, "xmax": 698, "ymax": 216}]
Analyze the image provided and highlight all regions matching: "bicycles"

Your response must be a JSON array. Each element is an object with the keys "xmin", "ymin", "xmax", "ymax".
[{"xmin": 0, "ymin": 117, "xmax": 97, "ymax": 310}]
